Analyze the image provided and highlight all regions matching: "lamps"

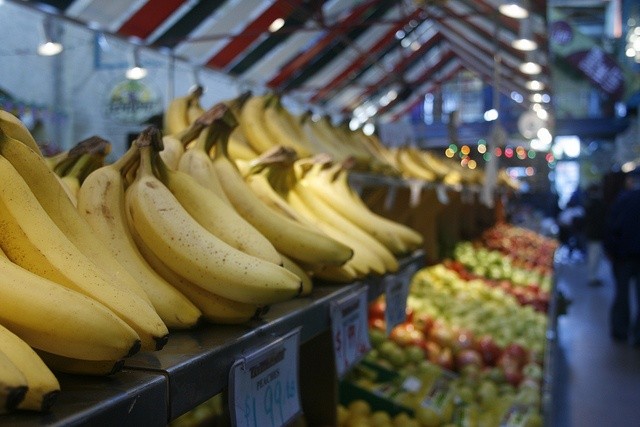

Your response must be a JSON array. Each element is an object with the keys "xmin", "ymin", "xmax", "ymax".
[
  {"xmin": 124, "ymin": 45, "xmax": 147, "ymax": 80},
  {"xmin": 35, "ymin": 15, "xmax": 63, "ymax": 57},
  {"xmin": 519, "ymin": 62, "xmax": 542, "ymax": 75},
  {"xmin": 512, "ymin": 37, "xmax": 538, "ymax": 52},
  {"xmin": 498, "ymin": 3, "xmax": 528, "ymax": 20}
]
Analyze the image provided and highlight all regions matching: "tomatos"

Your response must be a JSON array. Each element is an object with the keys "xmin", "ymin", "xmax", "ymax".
[{"xmin": 369, "ymin": 299, "xmax": 526, "ymax": 385}]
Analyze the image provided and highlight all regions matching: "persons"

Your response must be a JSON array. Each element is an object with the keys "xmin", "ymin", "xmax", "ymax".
[
  {"xmin": 563, "ymin": 193, "xmax": 586, "ymax": 263},
  {"xmin": 607, "ymin": 169, "xmax": 640, "ymax": 347},
  {"xmin": 585, "ymin": 182, "xmax": 606, "ymax": 286},
  {"xmin": 594, "ymin": 171, "xmax": 635, "ymax": 345}
]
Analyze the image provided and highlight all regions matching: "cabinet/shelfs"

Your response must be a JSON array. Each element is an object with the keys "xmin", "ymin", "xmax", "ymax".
[{"xmin": 29, "ymin": 249, "xmax": 424, "ymax": 426}]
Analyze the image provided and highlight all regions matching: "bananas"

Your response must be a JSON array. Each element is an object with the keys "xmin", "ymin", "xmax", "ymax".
[
  {"xmin": 244, "ymin": 144, "xmax": 400, "ymax": 283},
  {"xmin": 159, "ymin": 102, "xmax": 354, "ymax": 299},
  {"xmin": 76, "ymin": 124, "xmax": 305, "ymax": 331},
  {"xmin": 166, "ymin": 85, "xmax": 487, "ymax": 183},
  {"xmin": 46, "ymin": 135, "xmax": 111, "ymax": 198},
  {"xmin": 0, "ymin": 108, "xmax": 169, "ymax": 414},
  {"xmin": 294, "ymin": 153, "xmax": 424, "ymax": 257}
]
